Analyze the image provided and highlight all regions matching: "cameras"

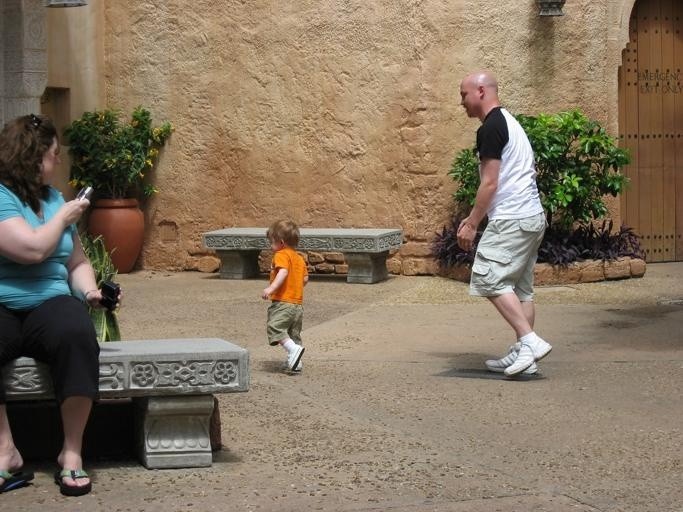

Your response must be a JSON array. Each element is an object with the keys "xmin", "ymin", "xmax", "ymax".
[{"xmin": 100, "ymin": 280, "xmax": 119, "ymax": 311}]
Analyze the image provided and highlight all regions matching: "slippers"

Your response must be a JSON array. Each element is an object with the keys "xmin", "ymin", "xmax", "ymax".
[
  {"xmin": 54, "ymin": 467, "xmax": 92, "ymax": 496},
  {"xmin": 0, "ymin": 469, "xmax": 34, "ymax": 494}
]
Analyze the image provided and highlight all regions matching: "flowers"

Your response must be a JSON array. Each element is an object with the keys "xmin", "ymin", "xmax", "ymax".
[{"xmin": 62, "ymin": 105, "xmax": 176, "ymax": 200}]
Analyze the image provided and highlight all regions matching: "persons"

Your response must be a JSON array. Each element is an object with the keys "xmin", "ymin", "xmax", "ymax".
[
  {"xmin": 456, "ymin": 71, "xmax": 552, "ymax": 378},
  {"xmin": 261, "ymin": 220, "xmax": 308, "ymax": 371},
  {"xmin": 0, "ymin": 113, "xmax": 124, "ymax": 497}
]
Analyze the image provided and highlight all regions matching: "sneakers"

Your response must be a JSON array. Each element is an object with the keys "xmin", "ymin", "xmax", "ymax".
[
  {"xmin": 295, "ymin": 359, "xmax": 303, "ymax": 371},
  {"xmin": 485, "ymin": 342, "xmax": 538, "ymax": 375},
  {"xmin": 504, "ymin": 332, "xmax": 552, "ymax": 377},
  {"xmin": 287, "ymin": 344, "xmax": 305, "ymax": 371}
]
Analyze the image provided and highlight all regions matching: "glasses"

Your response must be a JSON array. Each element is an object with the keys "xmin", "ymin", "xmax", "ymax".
[{"xmin": 30, "ymin": 114, "xmax": 42, "ymax": 134}]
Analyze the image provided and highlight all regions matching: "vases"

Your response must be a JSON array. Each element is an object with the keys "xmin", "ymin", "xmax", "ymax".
[{"xmin": 88, "ymin": 198, "xmax": 145, "ymax": 274}]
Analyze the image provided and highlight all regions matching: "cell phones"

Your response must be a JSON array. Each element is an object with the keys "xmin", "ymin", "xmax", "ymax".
[{"xmin": 75, "ymin": 185, "xmax": 94, "ymax": 201}]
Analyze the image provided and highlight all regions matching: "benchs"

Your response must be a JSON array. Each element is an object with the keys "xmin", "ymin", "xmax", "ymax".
[
  {"xmin": 2, "ymin": 338, "xmax": 249, "ymax": 470},
  {"xmin": 202, "ymin": 227, "xmax": 403, "ymax": 284}
]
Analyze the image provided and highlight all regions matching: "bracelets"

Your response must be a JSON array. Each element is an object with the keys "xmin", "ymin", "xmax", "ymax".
[{"xmin": 83, "ymin": 289, "xmax": 97, "ymax": 301}]
